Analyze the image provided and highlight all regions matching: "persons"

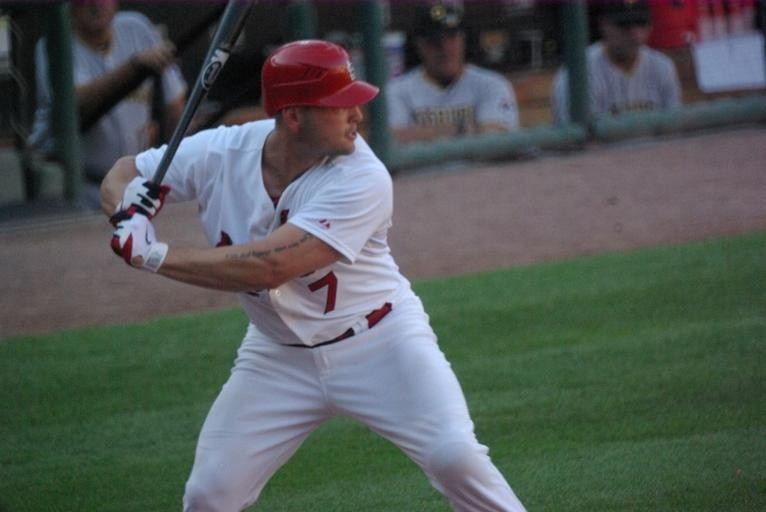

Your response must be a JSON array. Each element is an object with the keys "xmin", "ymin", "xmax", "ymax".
[
  {"xmin": 99, "ymin": 41, "xmax": 524, "ymax": 512},
  {"xmin": 199, "ymin": 1, "xmax": 766, "ymax": 152},
  {"xmin": 25, "ymin": 1, "xmax": 193, "ymax": 210}
]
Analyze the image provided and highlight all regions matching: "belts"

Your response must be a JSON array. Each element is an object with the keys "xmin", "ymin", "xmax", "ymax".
[{"xmin": 285, "ymin": 303, "xmax": 392, "ymax": 348}]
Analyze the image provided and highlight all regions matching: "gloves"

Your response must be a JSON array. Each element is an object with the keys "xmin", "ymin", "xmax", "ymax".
[
  {"xmin": 116, "ymin": 176, "xmax": 171, "ymax": 220},
  {"xmin": 108, "ymin": 206, "xmax": 169, "ymax": 275}
]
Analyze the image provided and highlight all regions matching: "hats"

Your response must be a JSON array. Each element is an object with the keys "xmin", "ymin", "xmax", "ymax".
[{"xmin": 412, "ymin": 3, "xmax": 464, "ymax": 35}]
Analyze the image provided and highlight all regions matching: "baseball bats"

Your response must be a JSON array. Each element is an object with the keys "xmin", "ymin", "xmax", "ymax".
[{"xmin": 151, "ymin": 0, "xmax": 255, "ymax": 185}]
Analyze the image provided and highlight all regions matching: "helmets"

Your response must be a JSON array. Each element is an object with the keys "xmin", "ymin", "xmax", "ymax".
[{"xmin": 262, "ymin": 39, "xmax": 380, "ymax": 119}]
